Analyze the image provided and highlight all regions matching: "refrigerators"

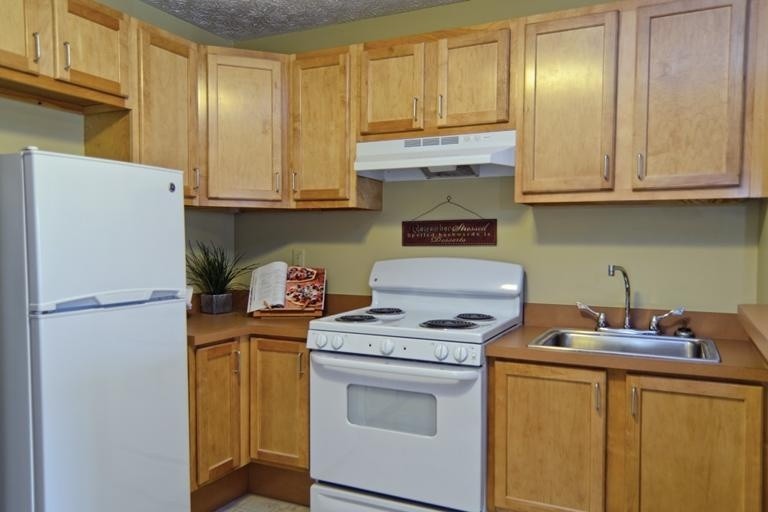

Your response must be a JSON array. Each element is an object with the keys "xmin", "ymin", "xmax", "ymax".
[{"xmin": 0, "ymin": 144, "xmax": 192, "ymax": 512}]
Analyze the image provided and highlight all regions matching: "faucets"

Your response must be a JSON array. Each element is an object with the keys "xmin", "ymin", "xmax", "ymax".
[{"xmin": 608, "ymin": 265, "xmax": 632, "ymax": 329}]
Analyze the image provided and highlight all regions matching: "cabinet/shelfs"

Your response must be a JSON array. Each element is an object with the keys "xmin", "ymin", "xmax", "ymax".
[
  {"xmin": 515, "ymin": 0, "xmax": 768, "ymax": 203},
  {"xmin": 188, "ymin": 336, "xmax": 248, "ymax": 512},
  {"xmin": 484, "ymin": 359, "xmax": 768, "ymax": 512},
  {"xmin": 84, "ymin": 19, "xmax": 199, "ymax": 207},
  {"xmin": 0, "ymin": 0, "xmax": 133, "ymax": 115},
  {"xmin": 248, "ymin": 335, "xmax": 311, "ymax": 508},
  {"xmin": 355, "ymin": 18, "xmax": 517, "ymax": 143},
  {"xmin": 198, "ymin": 44, "xmax": 383, "ymax": 211}
]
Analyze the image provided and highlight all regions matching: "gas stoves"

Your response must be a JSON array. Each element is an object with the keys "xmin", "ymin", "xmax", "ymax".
[{"xmin": 305, "ymin": 305, "xmax": 526, "ymax": 342}]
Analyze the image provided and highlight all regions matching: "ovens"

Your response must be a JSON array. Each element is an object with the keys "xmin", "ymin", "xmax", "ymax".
[{"xmin": 307, "ymin": 330, "xmax": 488, "ymax": 512}]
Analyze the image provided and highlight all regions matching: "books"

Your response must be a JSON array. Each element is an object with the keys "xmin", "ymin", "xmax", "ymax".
[{"xmin": 246, "ymin": 261, "xmax": 327, "ymax": 313}]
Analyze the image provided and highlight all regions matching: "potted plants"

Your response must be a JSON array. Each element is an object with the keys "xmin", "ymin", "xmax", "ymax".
[{"xmin": 185, "ymin": 238, "xmax": 260, "ymax": 314}]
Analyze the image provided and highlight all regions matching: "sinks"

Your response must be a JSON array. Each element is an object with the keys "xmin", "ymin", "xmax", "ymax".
[{"xmin": 543, "ymin": 333, "xmax": 700, "ymax": 358}]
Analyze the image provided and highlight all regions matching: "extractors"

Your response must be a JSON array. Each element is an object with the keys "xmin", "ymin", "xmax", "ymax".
[{"xmin": 351, "ymin": 131, "xmax": 520, "ymax": 183}]
